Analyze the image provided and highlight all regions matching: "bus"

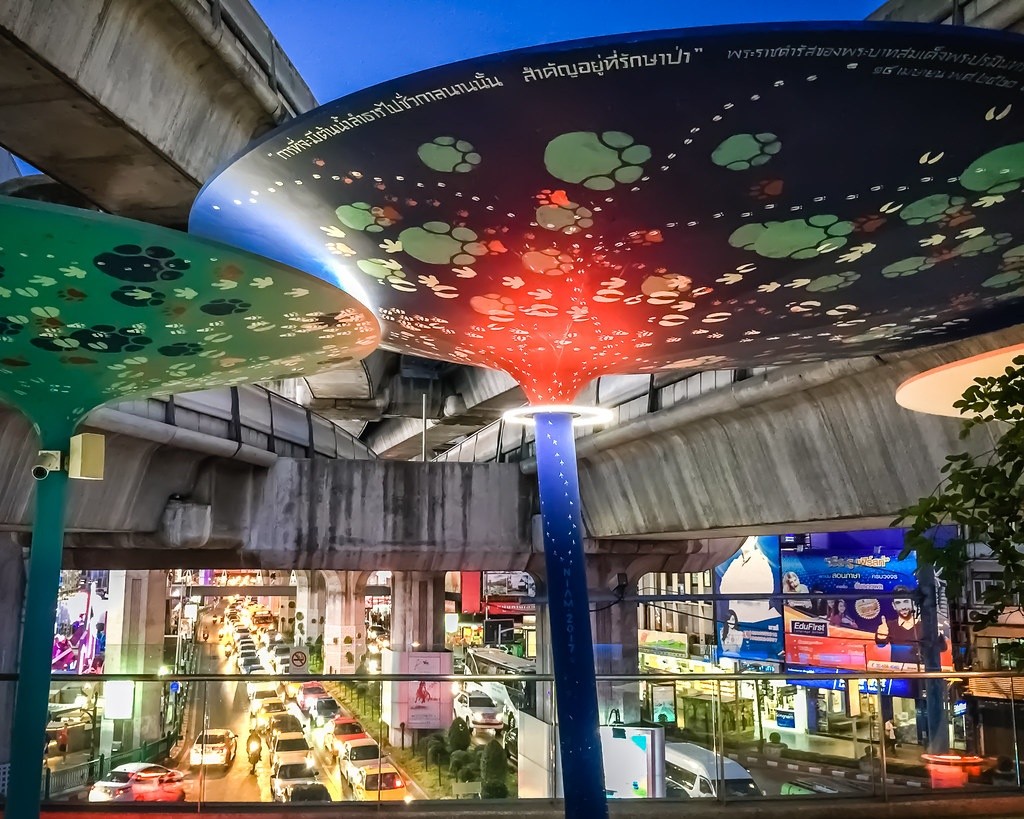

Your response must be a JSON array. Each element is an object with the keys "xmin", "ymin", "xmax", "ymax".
[{"xmin": 464, "ymin": 648, "xmax": 537, "ymax": 729}]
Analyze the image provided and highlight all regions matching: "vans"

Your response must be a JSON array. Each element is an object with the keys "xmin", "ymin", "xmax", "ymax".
[{"xmin": 664, "ymin": 742, "xmax": 763, "ymax": 798}]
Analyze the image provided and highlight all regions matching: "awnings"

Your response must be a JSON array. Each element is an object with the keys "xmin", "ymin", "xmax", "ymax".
[{"xmin": 973, "ymin": 624, "xmax": 1024, "ymax": 639}]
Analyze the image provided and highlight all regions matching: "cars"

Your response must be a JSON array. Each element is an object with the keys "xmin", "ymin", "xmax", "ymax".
[
  {"xmin": 88, "ymin": 763, "xmax": 185, "ymax": 802},
  {"xmin": 226, "ymin": 596, "xmax": 412, "ymax": 803},
  {"xmin": 453, "ymin": 691, "xmax": 504, "ymax": 733},
  {"xmin": 189, "ymin": 729, "xmax": 238, "ymax": 769}
]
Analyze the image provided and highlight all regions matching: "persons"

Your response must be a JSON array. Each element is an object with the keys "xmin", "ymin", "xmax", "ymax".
[
  {"xmin": 876, "ymin": 586, "xmax": 948, "ymax": 665},
  {"xmin": 43, "ymin": 732, "xmax": 51, "ymax": 766},
  {"xmin": 225, "ymin": 641, "xmax": 232, "ymax": 647},
  {"xmin": 451, "ymin": 633, "xmax": 482, "ymax": 655},
  {"xmin": 247, "ymin": 730, "xmax": 262, "ymax": 759},
  {"xmin": 885, "ymin": 718, "xmax": 897, "ymax": 755},
  {"xmin": 160, "ymin": 711, "xmax": 164, "ymax": 732},
  {"xmin": 783, "ymin": 573, "xmax": 862, "ymax": 630},
  {"xmin": 719, "ymin": 610, "xmax": 743, "ymax": 652},
  {"xmin": 57, "ymin": 723, "xmax": 69, "ymax": 765},
  {"xmin": 719, "ymin": 535, "xmax": 774, "ymax": 622},
  {"xmin": 371, "ymin": 608, "xmax": 390, "ymax": 629},
  {"xmin": 203, "ymin": 613, "xmax": 225, "ymax": 636}
]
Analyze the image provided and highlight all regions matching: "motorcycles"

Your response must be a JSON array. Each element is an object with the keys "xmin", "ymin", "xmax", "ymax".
[
  {"xmin": 246, "ymin": 742, "xmax": 262, "ymax": 774},
  {"xmin": 204, "ymin": 632, "xmax": 208, "ymax": 642}
]
